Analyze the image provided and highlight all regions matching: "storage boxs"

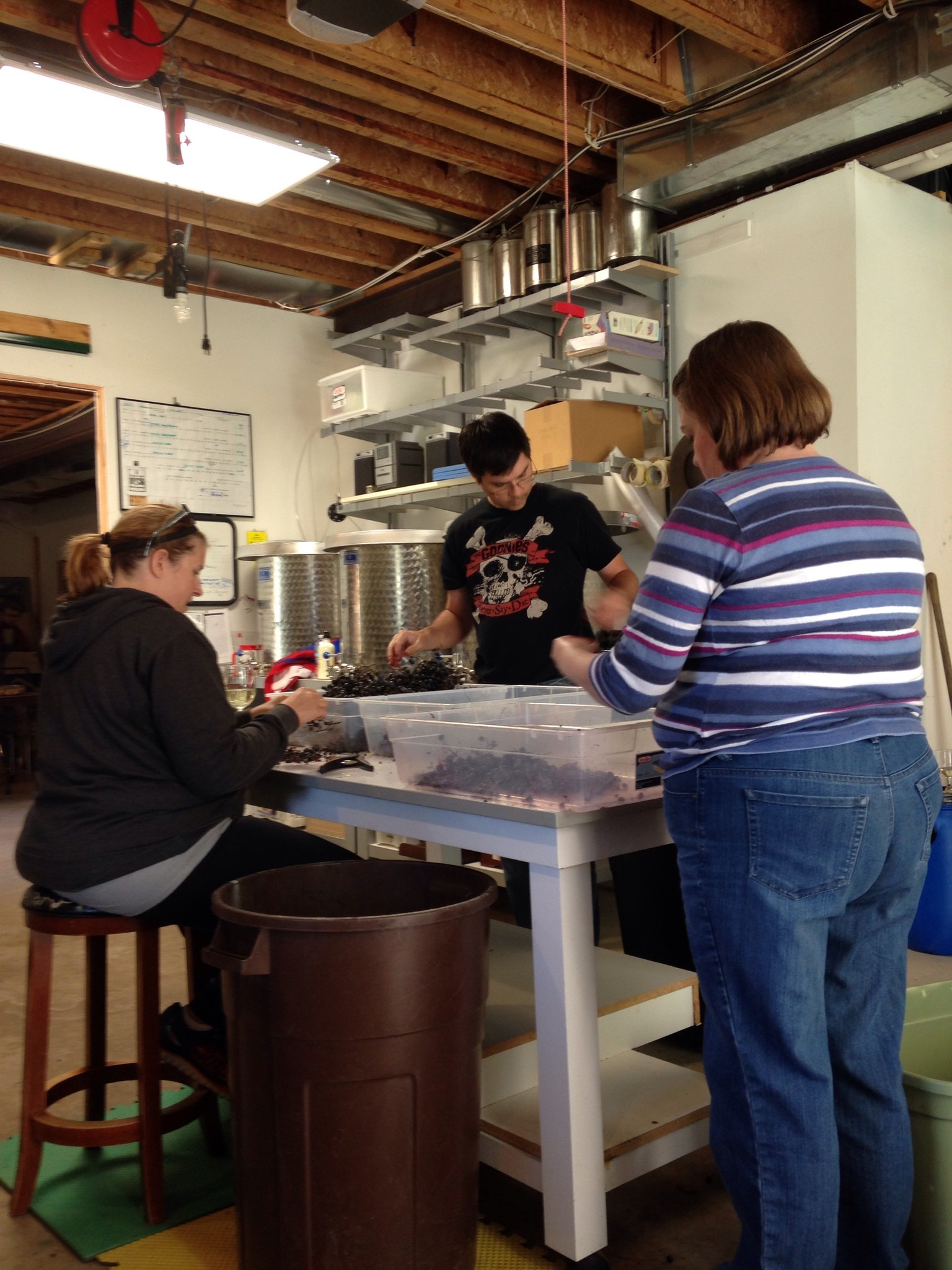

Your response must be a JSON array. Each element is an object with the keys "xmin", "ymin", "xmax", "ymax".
[
  {"xmin": 317, "ymin": 365, "xmax": 444, "ymax": 425},
  {"xmin": 524, "ymin": 398, "xmax": 645, "ymax": 473},
  {"xmin": 561, "ymin": 311, "xmax": 666, "ymax": 360},
  {"xmin": 265, "ymin": 683, "xmax": 664, "ymax": 814}
]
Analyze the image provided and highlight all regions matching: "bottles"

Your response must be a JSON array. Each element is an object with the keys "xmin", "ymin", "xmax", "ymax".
[
  {"xmin": 318, "ymin": 630, "xmax": 336, "ymax": 679},
  {"xmin": 314, "ymin": 634, "xmax": 323, "ymax": 677}
]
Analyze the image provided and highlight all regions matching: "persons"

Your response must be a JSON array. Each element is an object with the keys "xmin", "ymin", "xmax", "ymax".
[
  {"xmin": 551, "ymin": 320, "xmax": 944, "ymax": 1270},
  {"xmin": 387, "ymin": 412, "xmax": 639, "ymax": 950},
  {"xmin": 14, "ymin": 502, "xmax": 364, "ymax": 1100},
  {"xmin": 588, "ymin": 438, "xmax": 705, "ymax": 1052}
]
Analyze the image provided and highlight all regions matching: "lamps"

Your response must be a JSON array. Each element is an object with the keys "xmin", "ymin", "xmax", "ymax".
[{"xmin": 0, "ymin": 50, "xmax": 340, "ymax": 207}]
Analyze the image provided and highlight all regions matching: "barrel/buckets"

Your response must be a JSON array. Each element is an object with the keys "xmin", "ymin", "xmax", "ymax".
[
  {"xmin": 524, "ymin": 202, "xmax": 566, "ymax": 296},
  {"xmin": 601, "ymin": 182, "xmax": 660, "ymax": 268},
  {"xmin": 562, "ymin": 199, "xmax": 602, "ymax": 284},
  {"xmin": 459, "ymin": 239, "xmax": 498, "ymax": 317},
  {"xmin": 256, "ymin": 556, "xmax": 343, "ymax": 662},
  {"xmin": 494, "ymin": 235, "xmax": 525, "ymax": 309},
  {"xmin": 896, "ymin": 980, "xmax": 950, "ymax": 1270},
  {"xmin": 338, "ymin": 544, "xmax": 454, "ymax": 667}
]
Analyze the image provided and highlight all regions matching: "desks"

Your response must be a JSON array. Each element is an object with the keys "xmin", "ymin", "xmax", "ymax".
[{"xmin": 241, "ymin": 752, "xmax": 714, "ymax": 1263}]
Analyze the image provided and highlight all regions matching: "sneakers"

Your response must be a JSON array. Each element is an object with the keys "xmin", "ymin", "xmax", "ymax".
[{"xmin": 158, "ymin": 1003, "xmax": 228, "ymax": 1097}]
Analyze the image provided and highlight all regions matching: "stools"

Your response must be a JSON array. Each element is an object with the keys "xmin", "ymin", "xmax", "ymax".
[{"xmin": 9, "ymin": 884, "xmax": 228, "ymax": 1225}]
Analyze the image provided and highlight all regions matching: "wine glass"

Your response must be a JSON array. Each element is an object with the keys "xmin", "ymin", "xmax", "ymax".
[{"xmin": 225, "ymin": 664, "xmax": 256, "ymax": 711}]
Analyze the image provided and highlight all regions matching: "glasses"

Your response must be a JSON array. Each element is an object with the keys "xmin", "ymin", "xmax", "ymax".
[
  {"xmin": 144, "ymin": 503, "xmax": 196, "ymax": 557},
  {"xmin": 479, "ymin": 460, "xmax": 538, "ymax": 498}
]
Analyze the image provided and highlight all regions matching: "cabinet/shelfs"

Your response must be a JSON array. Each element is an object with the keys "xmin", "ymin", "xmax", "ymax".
[{"xmin": 319, "ymin": 232, "xmax": 681, "ymax": 521}]
